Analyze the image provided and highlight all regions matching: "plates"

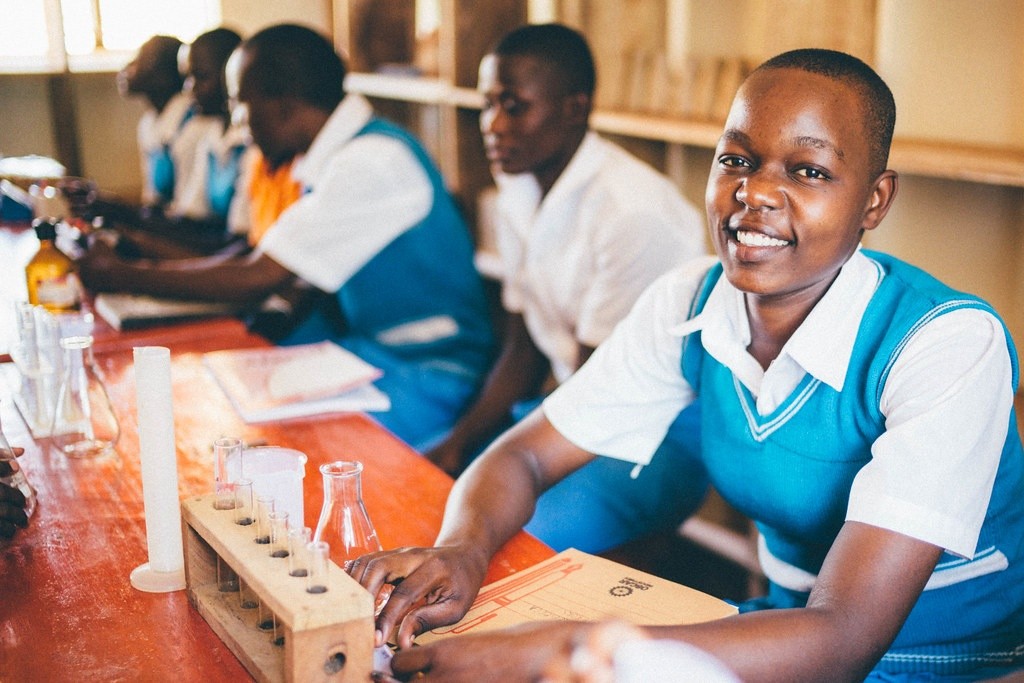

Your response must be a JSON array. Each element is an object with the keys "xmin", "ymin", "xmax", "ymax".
[{"xmin": 200, "ymin": 344, "xmax": 393, "ymax": 429}]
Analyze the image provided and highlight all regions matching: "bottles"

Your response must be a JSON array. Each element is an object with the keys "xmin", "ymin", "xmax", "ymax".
[
  {"xmin": 0, "ymin": 413, "xmax": 37, "ymax": 529},
  {"xmin": 25, "ymin": 220, "xmax": 83, "ymax": 314},
  {"xmin": 313, "ymin": 459, "xmax": 394, "ymax": 618}
]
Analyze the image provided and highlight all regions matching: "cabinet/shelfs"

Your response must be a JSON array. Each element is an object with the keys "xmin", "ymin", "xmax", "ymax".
[{"xmin": 332, "ymin": 0, "xmax": 1024, "ymax": 534}]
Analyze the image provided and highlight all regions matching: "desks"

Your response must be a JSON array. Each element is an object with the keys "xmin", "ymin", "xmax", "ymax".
[{"xmin": 0, "ymin": 184, "xmax": 559, "ymax": 683}]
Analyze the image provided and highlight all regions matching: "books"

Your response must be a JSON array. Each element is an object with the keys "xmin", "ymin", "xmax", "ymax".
[
  {"xmin": 408, "ymin": 547, "xmax": 738, "ymax": 650},
  {"xmin": 205, "ymin": 339, "xmax": 391, "ymax": 424}
]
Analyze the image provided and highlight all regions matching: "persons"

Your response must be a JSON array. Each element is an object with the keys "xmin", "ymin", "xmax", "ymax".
[
  {"xmin": 341, "ymin": 48, "xmax": 1023, "ymax": 683},
  {"xmin": 59, "ymin": 24, "xmax": 498, "ymax": 456},
  {"xmin": 420, "ymin": 24, "xmax": 711, "ymax": 556}
]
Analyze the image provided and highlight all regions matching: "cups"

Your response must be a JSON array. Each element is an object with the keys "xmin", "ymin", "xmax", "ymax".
[{"xmin": 225, "ymin": 445, "xmax": 307, "ymax": 534}]
